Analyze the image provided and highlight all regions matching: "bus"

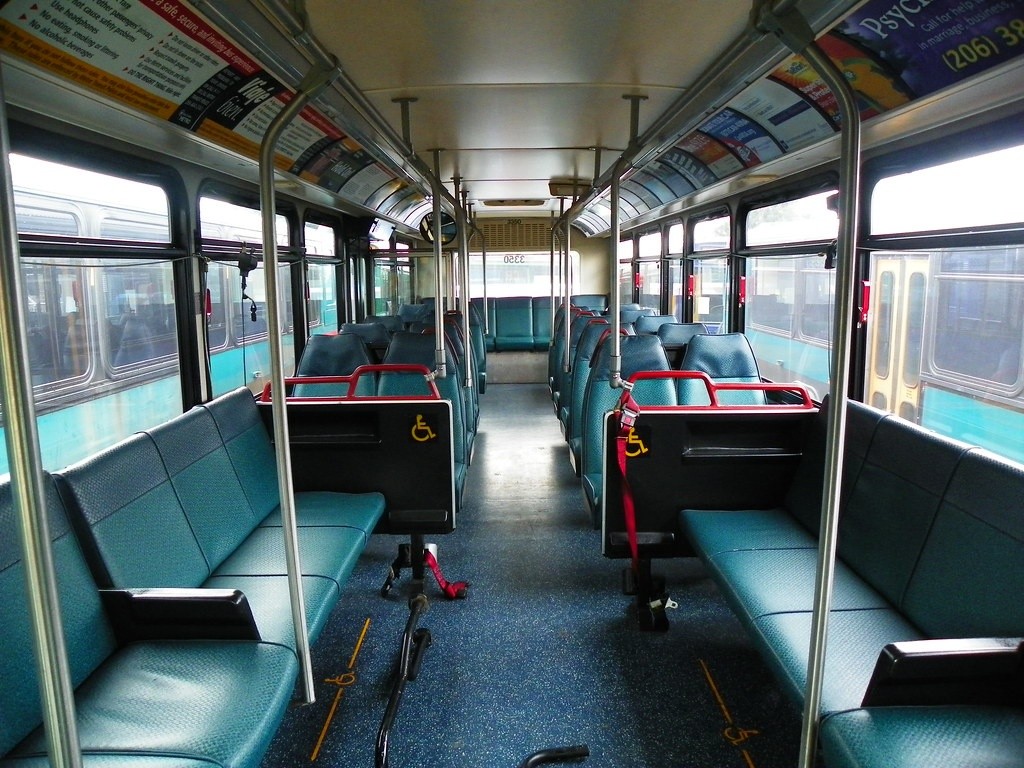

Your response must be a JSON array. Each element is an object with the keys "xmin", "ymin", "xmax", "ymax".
[
  {"xmin": 455, "ymin": 136, "xmax": 1023, "ymax": 478},
  {"xmin": 0, "ymin": 152, "xmax": 410, "ymax": 485}
]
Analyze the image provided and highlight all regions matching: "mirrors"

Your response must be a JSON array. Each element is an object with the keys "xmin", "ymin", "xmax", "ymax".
[{"xmin": 419, "ymin": 211, "xmax": 457, "ymax": 246}]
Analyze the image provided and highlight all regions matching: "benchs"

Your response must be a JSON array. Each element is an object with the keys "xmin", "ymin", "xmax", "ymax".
[{"xmin": 1, "ymin": 293, "xmax": 1024, "ymax": 768}]
